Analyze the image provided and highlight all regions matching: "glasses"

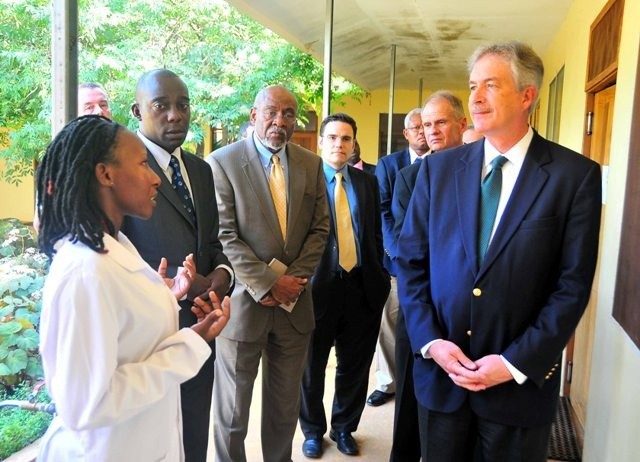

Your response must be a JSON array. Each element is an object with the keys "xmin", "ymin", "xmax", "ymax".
[
  {"xmin": 406, "ymin": 126, "xmax": 423, "ymax": 131},
  {"xmin": 322, "ymin": 133, "xmax": 352, "ymax": 143}
]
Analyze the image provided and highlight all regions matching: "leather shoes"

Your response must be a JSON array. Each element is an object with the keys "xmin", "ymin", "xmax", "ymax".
[
  {"xmin": 302, "ymin": 437, "xmax": 323, "ymax": 457},
  {"xmin": 329, "ymin": 430, "xmax": 358, "ymax": 455},
  {"xmin": 367, "ymin": 390, "xmax": 395, "ymax": 406}
]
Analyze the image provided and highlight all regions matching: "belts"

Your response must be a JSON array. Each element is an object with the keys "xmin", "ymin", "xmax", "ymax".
[{"xmin": 335, "ymin": 270, "xmax": 359, "ymax": 280}]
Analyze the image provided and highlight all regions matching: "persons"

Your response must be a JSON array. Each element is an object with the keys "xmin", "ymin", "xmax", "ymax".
[
  {"xmin": 299, "ymin": 113, "xmax": 390, "ymax": 458},
  {"xmin": 390, "ymin": 90, "xmax": 467, "ymax": 462},
  {"xmin": 35, "ymin": 117, "xmax": 231, "ymax": 462},
  {"xmin": 347, "ymin": 137, "xmax": 377, "ymax": 175},
  {"xmin": 203, "ymin": 86, "xmax": 330, "ymax": 462},
  {"xmin": 367, "ymin": 107, "xmax": 437, "ymax": 406},
  {"xmin": 122, "ymin": 68, "xmax": 235, "ymax": 462},
  {"xmin": 398, "ymin": 43, "xmax": 602, "ymax": 462},
  {"xmin": 461, "ymin": 125, "xmax": 483, "ymax": 145},
  {"xmin": 77, "ymin": 81, "xmax": 111, "ymax": 117}
]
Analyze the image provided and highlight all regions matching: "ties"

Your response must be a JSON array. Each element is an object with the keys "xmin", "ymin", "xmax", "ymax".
[
  {"xmin": 478, "ymin": 156, "xmax": 509, "ymax": 275},
  {"xmin": 170, "ymin": 154, "xmax": 197, "ymax": 232},
  {"xmin": 269, "ymin": 155, "xmax": 287, "ymax": 242},
  {"xmin": 333, "ymin": 172, "xmax": 358, "ymax": 273}
]
